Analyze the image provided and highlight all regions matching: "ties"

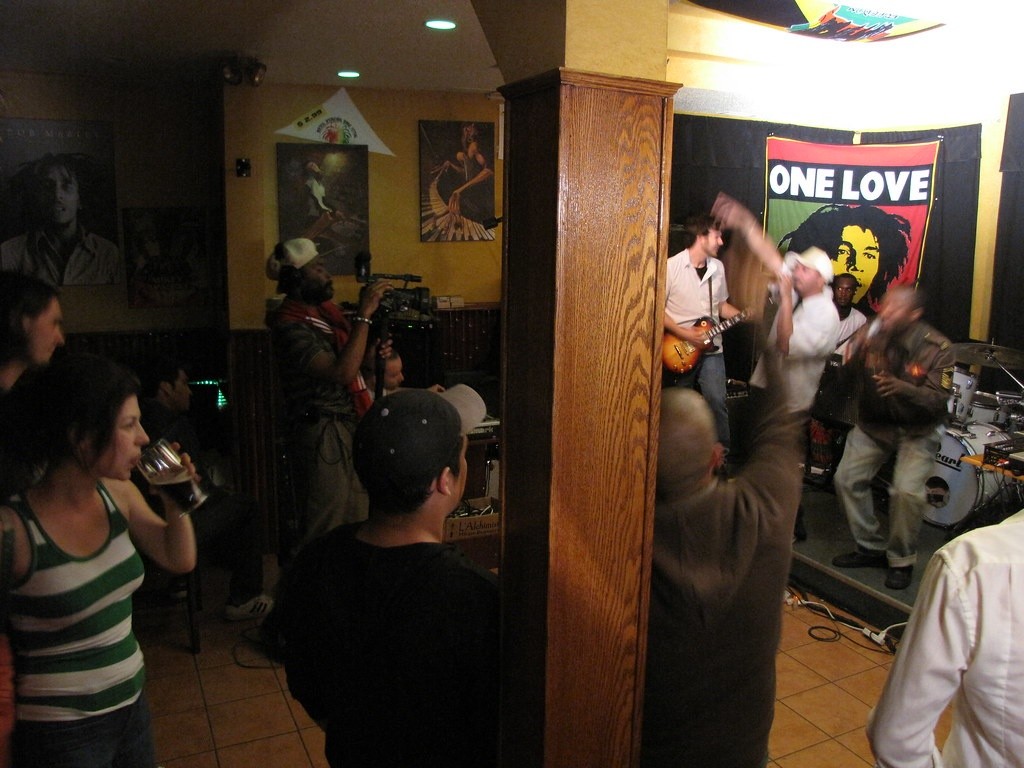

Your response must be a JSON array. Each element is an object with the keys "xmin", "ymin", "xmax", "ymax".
[{"xmin": 792, "ymin": 294, "xmax": 802, "ymax": 315}]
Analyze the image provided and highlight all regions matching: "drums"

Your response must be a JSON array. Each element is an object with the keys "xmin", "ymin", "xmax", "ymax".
[
  {"xmin": 920, "ymin": 421, "xmax": 1014, "ymax": 530},
  {"xmin": 946, "ymin": 366, "xmax": 979, "ymax": 424},
  {"xmin": 966, "ymin": 391, "xmax": 1006, "ymax": 425}
]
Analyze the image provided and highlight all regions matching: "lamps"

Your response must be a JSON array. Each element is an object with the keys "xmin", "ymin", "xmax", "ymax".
[{"xmin": 221, "ymin": 55, "xmax": 265, "ymax": 86}]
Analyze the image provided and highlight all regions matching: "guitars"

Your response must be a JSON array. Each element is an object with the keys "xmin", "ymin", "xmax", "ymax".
[{"xmin": 662, "ymin": 305, "xmax": 756, "ymax": 376}]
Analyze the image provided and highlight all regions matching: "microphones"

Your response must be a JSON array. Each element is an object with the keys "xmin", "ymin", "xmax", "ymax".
[{"xmin": 373, "ymin": 272, "xmax": 422, "ymax": 283}]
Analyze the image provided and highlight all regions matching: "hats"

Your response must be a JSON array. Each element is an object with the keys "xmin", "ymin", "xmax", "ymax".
[
  {"xmin": 352, "ymin": 382, "xmax": 487, "ymax": 489},
  {"xmin": 791, "ymin": 247, "xmax": 834, "ymax": 283},
  {"xmin": 265, "ymin": 238, "xmax": 319, "ymax": 280}
]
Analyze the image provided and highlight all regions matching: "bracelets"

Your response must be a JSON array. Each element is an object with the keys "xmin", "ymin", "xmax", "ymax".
[{"xmin": 352, "ymin": 316, "xmax": 372, "ymax": 325}]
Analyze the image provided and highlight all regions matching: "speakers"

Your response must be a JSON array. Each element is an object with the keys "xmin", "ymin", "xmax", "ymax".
[{"xmin": 726, "ymin": 388, "xmax": 751, "ymax": 463}]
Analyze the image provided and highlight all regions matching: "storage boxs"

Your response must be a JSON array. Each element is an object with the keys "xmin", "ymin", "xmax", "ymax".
[{"xmin": 443, "ymin": 495, "xmax": 499, "ymax": 542}]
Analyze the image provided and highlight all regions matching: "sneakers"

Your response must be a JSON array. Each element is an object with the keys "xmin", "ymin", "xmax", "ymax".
[{"xmin": 227, "ymin": 592, "xmax": 275, "ymax": 622}]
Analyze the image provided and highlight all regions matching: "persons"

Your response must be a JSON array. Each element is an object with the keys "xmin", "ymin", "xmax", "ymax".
[
  {"xmin": 266, "ymin": 238, "xmax": 405, "ymax": 543},
  {"xmin": 284, "ymin": 383, "xmax": 498, "ymax": 768},
  {"xmin": 0, "ymin": 271, "xmax": 65, "ymax": 395},
  {"xmin": 666, "ymin": 214, "xmax": 755, "ymax": 450},
  {"xmin": 0, "ymin": 153, "xmax": 120, "ymax": 285},
  {"xmin": 711, "ymin": 192, "xmax": 841, "ymax": 413},
  {"xmin": 867, "ymin": 508, "xmax": 1024, "ymax": 768},
  {"xmin": 832, "ymin": 286, "xmax": 955, "ymax": 590},
  {"xmin": 0, "ymin": 352, "xmax": 198, "ymax": 768},
  {"xmin": 641, "ymin": 340, "xmax": 805, "ymax": 768},
  {"xmin": 138, "ymin": 363, "xmax": 275, "ymax": 622},
  {"xmin": 830, "ymin": 273, "xmax": 867, "ymax": 341}
]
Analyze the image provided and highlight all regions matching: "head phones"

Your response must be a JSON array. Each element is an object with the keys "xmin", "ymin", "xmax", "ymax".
[{"xmin": 274, "ymin": 243, "xmax": 302, "ymax": 293}]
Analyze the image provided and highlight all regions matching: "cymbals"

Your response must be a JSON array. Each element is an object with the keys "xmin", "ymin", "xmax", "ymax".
[{"xmin": 950, "ymin": 341, "xmax": 1024, "ymax": 370}]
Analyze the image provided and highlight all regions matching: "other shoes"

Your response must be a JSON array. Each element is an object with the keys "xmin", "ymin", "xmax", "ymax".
[
  {"xmin": 885, "ymin": 565, "xmax": 913, "ymax": 589},
  {"xmin": 832, "ymin": 552, "xmax": 889, "ymax": 569}
]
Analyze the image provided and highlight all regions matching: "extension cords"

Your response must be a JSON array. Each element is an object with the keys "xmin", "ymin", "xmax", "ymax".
[{"xmin": 863, "ymin": 628, "xmax": 885, "ymax": 645}]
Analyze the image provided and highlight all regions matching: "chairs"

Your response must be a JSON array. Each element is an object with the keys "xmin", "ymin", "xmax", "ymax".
[{"xmin": 132, "ymin": 493, "xmax": 205, "ymax": 656}]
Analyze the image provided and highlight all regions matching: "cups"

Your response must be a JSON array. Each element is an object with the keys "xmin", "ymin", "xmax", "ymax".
[{"xmin": 135, "ymin": 439, "xmax": 208, "ymax": 522}]
